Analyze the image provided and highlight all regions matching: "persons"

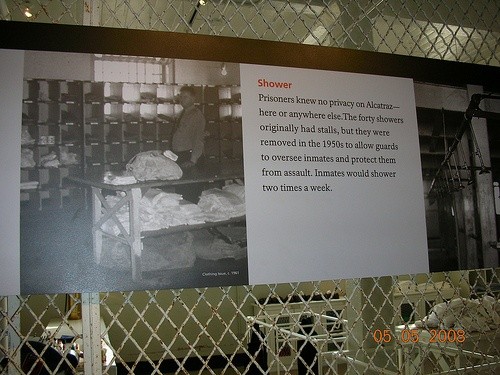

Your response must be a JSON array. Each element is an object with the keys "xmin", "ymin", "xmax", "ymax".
[{"xmin": 169, "ymin": 87, "xmax": 207, "ymax": 202}]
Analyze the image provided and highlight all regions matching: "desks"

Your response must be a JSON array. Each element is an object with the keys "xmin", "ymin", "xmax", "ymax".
[{"xmin": 64, "ymin": 161, "xmax": 247, "ymax": 286}]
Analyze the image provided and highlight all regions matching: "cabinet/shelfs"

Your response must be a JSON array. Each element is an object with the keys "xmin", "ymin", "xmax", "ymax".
[
  {"xmin": 249, "ymin": 297, "xmax": 352, "ymax": 373},
  {"xmin": 20, "ymin": 77, "xmax": 244, "ymax": 216},
  {"xmin": 392, "ymin": 287, "xmax": 463, "ymax": 327}
]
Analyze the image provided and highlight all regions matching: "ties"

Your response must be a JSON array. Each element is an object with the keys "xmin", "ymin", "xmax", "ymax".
[{"xmin": 176, "ymin": 110, "xmax": 185, "ymax": 131}]
